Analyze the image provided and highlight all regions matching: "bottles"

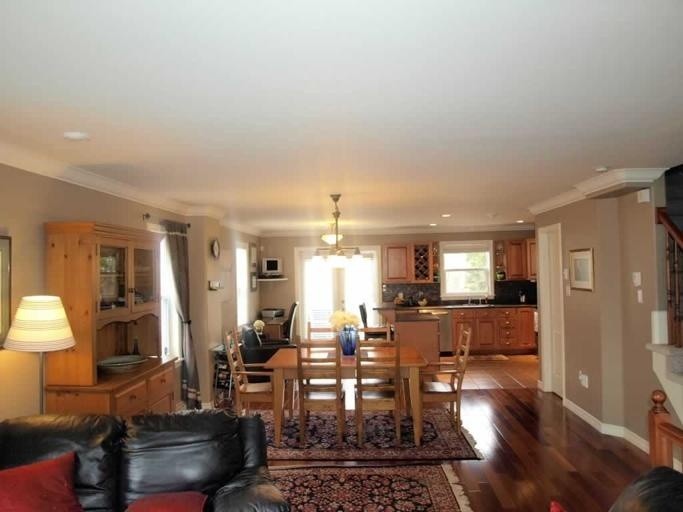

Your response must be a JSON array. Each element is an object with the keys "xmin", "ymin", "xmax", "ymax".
[
  {"xmin": 408, "ymin": 296, "xmax": 413, "ymax": 306},
  {"xmin": 130, "ymin": 339, "xmax": 141, "ymax": 356}
]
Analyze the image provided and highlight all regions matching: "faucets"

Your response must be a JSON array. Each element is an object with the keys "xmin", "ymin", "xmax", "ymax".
[
  {"xmin": 485, "ymin": 295, "xmax": 489, "ymax": 304},
  {"xmin": 468, "ymin": 296, "xmax": 472, "ymax": 304}
]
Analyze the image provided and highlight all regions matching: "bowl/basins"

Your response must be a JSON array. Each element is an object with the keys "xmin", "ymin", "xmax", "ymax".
[
  {"xmin": 95, "ymin": 354, "xmax": 147, "ymax": 376},
  {"xmin": 417, "ymin": 301, "xmax": 426, "ymax": 306},
  {"xmin": 101, "ymin": 256, "xmax": 115, "ymax": 273}
]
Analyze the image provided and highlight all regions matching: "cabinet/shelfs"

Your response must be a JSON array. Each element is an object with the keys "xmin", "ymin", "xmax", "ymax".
[
  {"xmin": 44, "ymin": 220, "xmax": 178, "ymax": 424},
  {"xmin": 452, "ymin": 307, "xmax": 536, "ymax": 355},
  {"xmin": 495, "ymin": 238, "xmax": 537, "ymax": 282},
  {"xmin": 383, "ymin": 241, "xmax": 440, "ymax": 284}
]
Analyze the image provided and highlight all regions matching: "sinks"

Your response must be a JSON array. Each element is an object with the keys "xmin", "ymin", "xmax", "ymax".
[{"xmin": 446, "ymin": 304, "xmax": 494, "ymax": 308}]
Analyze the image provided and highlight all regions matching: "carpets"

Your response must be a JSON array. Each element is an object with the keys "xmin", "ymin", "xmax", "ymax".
[
  {"xmin": 268, "ymin": 464, "xmax": 474, "ymax": 512},
  {"xmin": 242, "ymin": 408, "xmax": 485, "ymax": 461}
]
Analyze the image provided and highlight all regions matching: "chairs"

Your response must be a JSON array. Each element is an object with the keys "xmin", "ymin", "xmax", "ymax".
[
  {"xmin": 261, "ymin": 302, "xmax": 298, "ymax": 344},
  {"xmin": 359, "ymin": 303, "xmax": 394, "ymax": 341},
  {"xmin": 224, "ymin": 321, "xmax": 472, "ymax": 446}
]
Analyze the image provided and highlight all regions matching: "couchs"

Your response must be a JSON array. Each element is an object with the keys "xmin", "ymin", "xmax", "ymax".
[{"xmin": 0, "ymin": 407, "xmax": 291, "ymax": 512}]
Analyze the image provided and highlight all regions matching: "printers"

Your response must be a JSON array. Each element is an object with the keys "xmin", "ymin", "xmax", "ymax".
[{"xmin": 262, "ymin": 309, "xmax": 285, "ymax": 320}]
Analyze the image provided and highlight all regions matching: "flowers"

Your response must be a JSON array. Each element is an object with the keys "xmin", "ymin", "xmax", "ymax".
[{"xmin": 329, "ymin": 310, "xmax": 360, "ymax": 353}]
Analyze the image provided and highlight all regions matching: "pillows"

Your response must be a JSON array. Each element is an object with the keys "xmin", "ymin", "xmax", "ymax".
[
  {"xmin": 117, "ymin": 408, "xmax": 243, "ymax": 508},
  {"xmin": 608, "ymin": 467, "xmax": 683, "ymax": 512},
  {"xmin": 0, "ymin": 414, "xmax": 123, "ymax": 509},
  {"xmin": 124, "ymin": 490, "xmax": 209, "ymax": 512},
  {"xmin": 0, "ymin": 451, "xmax": 84, "ymax": 512}
]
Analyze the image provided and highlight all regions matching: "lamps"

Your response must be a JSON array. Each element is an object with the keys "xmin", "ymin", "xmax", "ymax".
[
  {"xmin": 320, "ymin": 223, "xmax": 343, "ymax": 245},
  {"xmin": 311, "ymin": 194, "xmax": 364, "ymax": 269},
  {"xmin": 3, "ymin": 295, "xmax": 78, "ymax": 414}
]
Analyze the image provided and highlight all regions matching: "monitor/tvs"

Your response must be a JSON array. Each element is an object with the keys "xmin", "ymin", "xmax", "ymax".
[{"xmin": 262, "ymin": 258, "xmax": 284, "ymax": 277}]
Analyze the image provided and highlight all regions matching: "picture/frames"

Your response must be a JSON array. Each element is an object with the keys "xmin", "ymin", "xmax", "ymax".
[
  {"xmin": 569, "ymin": 247, "xmax": 594, "ymax": 292},
  {"xmin": 250, "ymin": 272, "xmax": 257, "ymax": 291},
  {"xmin": 0, "ymin": 236, "xmax": 11, "ymax": 350},
  {"xmin": 249, "ymin": 242, "xmax": 256, "ymax": 267}
]
